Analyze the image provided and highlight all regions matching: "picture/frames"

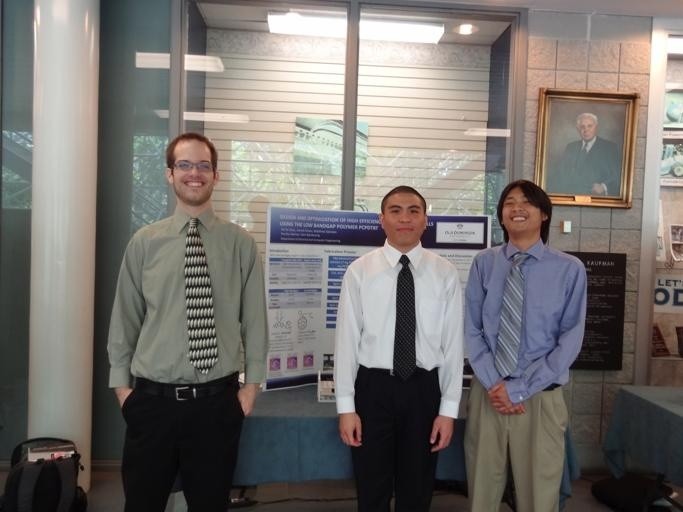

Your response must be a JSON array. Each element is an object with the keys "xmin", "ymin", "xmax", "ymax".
[{"xmin": 533, "ymin": 87, "xmax": 641, "ymax": 209}]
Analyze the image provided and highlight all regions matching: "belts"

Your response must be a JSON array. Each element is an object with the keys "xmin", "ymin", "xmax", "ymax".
[
  {"xmin": 136, "ymin": 378, "xmax": 236, "ymax": 402},
  {"xmin": 503, "ymin": 376, "xmax": 561, "ymax": 392}
]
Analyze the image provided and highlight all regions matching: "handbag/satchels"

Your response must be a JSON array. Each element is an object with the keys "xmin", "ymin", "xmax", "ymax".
[{"xmin": 592, "ymin": 472, "xmax": 676, "ymax": 512}]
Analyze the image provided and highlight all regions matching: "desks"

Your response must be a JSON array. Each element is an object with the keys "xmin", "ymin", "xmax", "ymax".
[
  {"xmin": 601, "ymin": 382, "xmax": 683, "ymax": 512},
  {"xmin": 229, "ymin": 385, "xmax": 570, "ymax": 508}
]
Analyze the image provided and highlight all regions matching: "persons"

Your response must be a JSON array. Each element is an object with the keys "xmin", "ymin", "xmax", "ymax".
[
  {"xmin": 333, "ymin": 184, "xmax": 464, "ymax": 512},
  {"xmin": 106, "ymin": 132, "xmax": 271, "ymax": 511},
  {"xmin": 462, "ymin": 180, "xmax": 588, "ymax": 512},
  {"xmin": 561, "ymin": 112, "xmax": 620, "ymax": 197}
]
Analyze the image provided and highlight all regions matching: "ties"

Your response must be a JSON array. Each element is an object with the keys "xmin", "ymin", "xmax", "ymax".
[
  {"xmin": 394, "ymin": 255, "xmax": 416, "ymax": 379},
  {"xmin": 494, "ymin": 253, "xmax": 528, "ymax": 378},
  {"xmin": 581, "ymin": 143, "xmax": 588, "ymax": 155},
  {"xmin": 183, "ymin": 218, "xmax": 218, "ymax": 374}
]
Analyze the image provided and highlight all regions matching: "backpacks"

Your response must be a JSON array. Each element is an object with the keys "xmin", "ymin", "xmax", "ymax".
[{"xmin": 0, "ymin": 438, "xmax": 87, "ymax": 512}]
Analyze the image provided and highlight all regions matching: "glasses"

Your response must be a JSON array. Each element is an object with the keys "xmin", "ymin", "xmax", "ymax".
[{"xmin": 173, "ymin": 160, "xmax": 212, "ymax": 171}]
